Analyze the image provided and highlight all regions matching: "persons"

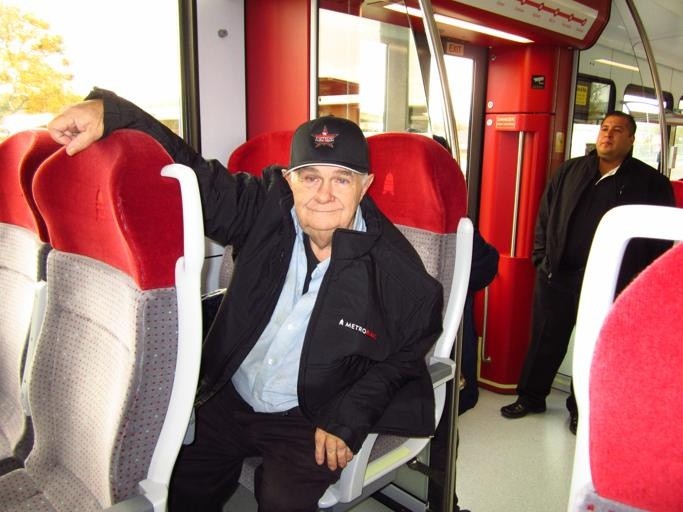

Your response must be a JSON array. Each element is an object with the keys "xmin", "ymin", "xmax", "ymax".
[
  {"xmin": 500, "ymin": 110, "xmax": 677, "ymax": 435},
  {"xmin": 403, "ymin": 128, "xmax": 502, "ymax": 512},
  {"xmin": 47, "ymin": 85, "xmax": 445, "ymax": 512}
]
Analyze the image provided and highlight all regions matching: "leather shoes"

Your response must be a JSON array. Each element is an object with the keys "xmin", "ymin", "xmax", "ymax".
[
  {"xmin": 566, "ymin": 395, "xmax": 578, "ymax": 435},
  {"xmin": 501, "ymin": 393, "xmax": 547, "ymax": 419}
]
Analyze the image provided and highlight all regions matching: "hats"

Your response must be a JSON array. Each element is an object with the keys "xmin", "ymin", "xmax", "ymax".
[{"xmin": 283, "ymin": 114, "xmax": 369, "ymax": 177}]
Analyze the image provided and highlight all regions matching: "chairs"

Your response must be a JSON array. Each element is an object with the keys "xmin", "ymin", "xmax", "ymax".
[
  {"xmin": 564, "ymin": 197, "xmax": 683, "ymax": 512},
  {"xmin": 201, "ymin": 126, "xmax": 293, "ymax": 305},
  {"xmin": 3, "ymin": 128, "xmax": 70, "ymax": 476},
  {"xmin": 237, "ymin": 130, "xmax": 476, "ymax": 511},
  {"xmin": 1, "ymin": 128, "xmax": 205, "ymax": 509}
]
